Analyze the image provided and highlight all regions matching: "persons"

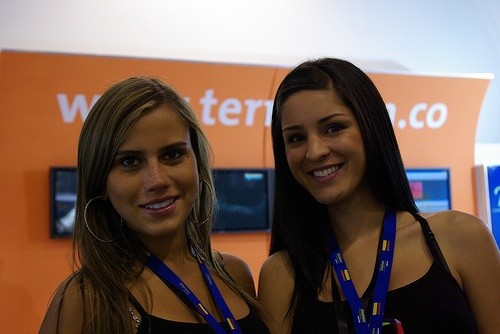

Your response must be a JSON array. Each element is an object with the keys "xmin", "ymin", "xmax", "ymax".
[
  {"xmin": 31, "ymin": 75, "xmax": 272, "ymax": 334},
  {"xmin": 257, "ymin": 57, "xmax": 499, "ymax": 334}
]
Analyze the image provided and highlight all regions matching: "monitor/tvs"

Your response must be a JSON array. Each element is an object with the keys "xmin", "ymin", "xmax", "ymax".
[
  {"xmin": 51, "ymin": 167, "xmax": 78, "ymax": 236},
  {"xmin": 205, "ymin": 168, "xmax": 273, "ymax": 234},
  {"xmin": 403, "ymin": 169, "xmax": 450, "ymax": 213}
]
